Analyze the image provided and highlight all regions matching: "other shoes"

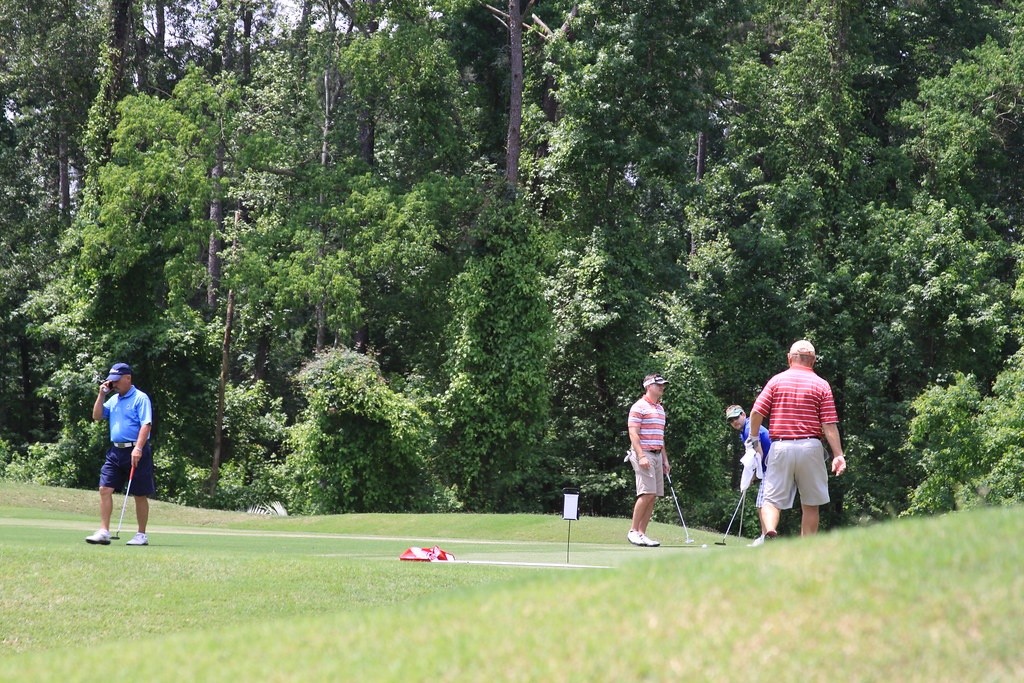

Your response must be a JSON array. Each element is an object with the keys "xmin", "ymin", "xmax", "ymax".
[{"xmin": 747, "ymin": 534, "xmax": 765, "ymax": 547}]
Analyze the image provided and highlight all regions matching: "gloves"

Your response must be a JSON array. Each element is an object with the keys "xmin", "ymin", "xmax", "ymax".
[
  {"xmin": 744, "ymin": 435, "xmax": 759, "ymax": 452},
  {"xmin": 624, "ymin": 450, "xmax": 631, "ymax": 463}
]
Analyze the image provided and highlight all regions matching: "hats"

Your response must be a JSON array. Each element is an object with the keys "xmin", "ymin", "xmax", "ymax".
[
  {"xmin": 643, "ymin": 376, "xmax": 669, "ymax": 388},
  {"xmin": 789, "ymin": 340, "xmax": 816, "ymax": 356},
  {"xmin": 725, "ymin": 405, "xmax": 744, "ymax": 419},
  {"xmin": 106, "ymin": 363, "xmax": 131, "ymax": 381}
]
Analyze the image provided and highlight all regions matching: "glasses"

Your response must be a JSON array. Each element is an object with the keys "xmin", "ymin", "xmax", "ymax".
[{"xmin": 727, "ymin": 414, "xmax": 740, "ymax": 423}]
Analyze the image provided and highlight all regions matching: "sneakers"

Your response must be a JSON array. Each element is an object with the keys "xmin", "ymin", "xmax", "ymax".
[
  {"xmin": 627, "ymin": 530, "xmax": 647, "ymax": 546},
  {"xmin": 126, "ymin": 533, "xmax": 148, "ymax": 545},
  {"xmin": 640, "ymin": 533, "xmax": 660, "ymax": 547},
  {"xmin": 85, "ymin": 530, "xmax": 110, "ymax": 545}
]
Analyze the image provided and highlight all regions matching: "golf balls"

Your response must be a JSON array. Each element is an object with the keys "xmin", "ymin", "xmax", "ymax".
[{"xmin": 702, "ymin": 544, "xmax": 707, "ymax": 549}]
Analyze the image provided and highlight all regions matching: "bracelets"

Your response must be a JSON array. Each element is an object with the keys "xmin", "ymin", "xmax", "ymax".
[
  {"xmin": 134, "ymin": 447, "xmax": 142, "ymax": 452},
  {"xmin": 637, "ymin": 455, "xmax": 645, "ymax": 461}
]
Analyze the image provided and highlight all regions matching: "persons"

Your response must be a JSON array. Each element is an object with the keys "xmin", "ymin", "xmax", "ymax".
[
  {"xmin": 726, "ymin": 406, "xmax": 777, "ymax": 549},
  {"xmin": 749, "ymin": 340, "xmax": 847, "ymax": 548},
  {"xmin": 85, "ymin": 363, "xmax": 152, "ymax": 546},
  {"xmin": 627, "ymin": 374, "xmax": 670, "ymax": 547}
]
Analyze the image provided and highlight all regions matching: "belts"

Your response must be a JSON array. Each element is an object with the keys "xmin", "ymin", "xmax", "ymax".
[
  {"xmin": 114, "ymin": 442, "xmax": 136, "ymax": 448},
  {"xmin": 650, "ymin": 450, "xmax": 661, "ymax": 454},
  {"xmin": 772, "ymin": 436, "xmax": 821, "ymax": 440}
]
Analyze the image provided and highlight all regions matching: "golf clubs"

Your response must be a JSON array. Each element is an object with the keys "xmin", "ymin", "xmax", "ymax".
[
  {"xmin": 665, "ymin": 471, "xmax": 695, "ymax": 544},
  {"xmin": 714, "ymin": 495, "xmax": 744, "ymax": 546},
  {"xmin": 738, "ymin": 490, "xmax": 746, "ymax": 542},
  {"xmin": 109, "ymin": 464, "xmax": 135, "ymax": 540}
]
